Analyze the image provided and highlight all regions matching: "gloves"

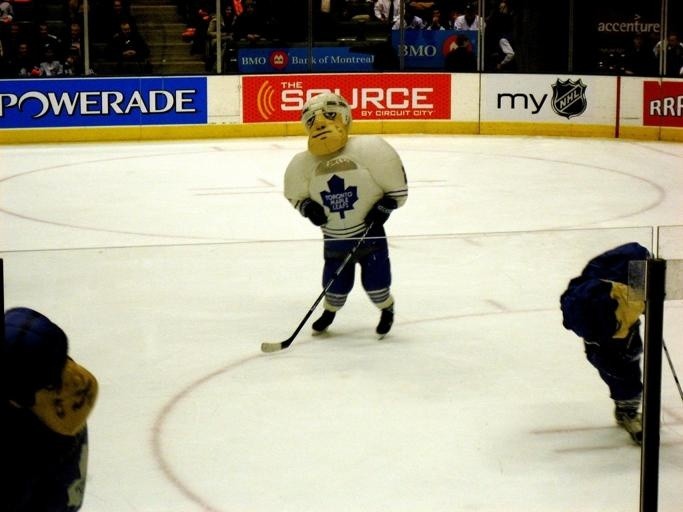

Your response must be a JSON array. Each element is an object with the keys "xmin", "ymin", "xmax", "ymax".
[
  {"xmin": 299, "ymin": 197, "xmax": 328, "ymax": 225},
  {"xmin": 365, "ymin": 194, "xmax": 398, "ymax": 233}
]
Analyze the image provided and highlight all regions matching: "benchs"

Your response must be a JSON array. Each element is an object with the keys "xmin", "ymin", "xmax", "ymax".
[{"xmin": 13, "ymin": 0, "xmax": 207, "ymax": 76}]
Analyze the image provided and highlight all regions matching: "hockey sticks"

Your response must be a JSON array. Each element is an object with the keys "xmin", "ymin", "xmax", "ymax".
[{"xmin": 261, "ymin": 218, "xmax": 376, "ymax": 353}]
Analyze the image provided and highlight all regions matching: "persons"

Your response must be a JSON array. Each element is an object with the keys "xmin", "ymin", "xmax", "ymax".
[
  {"xmin": 179, "ymin": 1, "xmax": 683, "ymax": 77},
  {"xmin": 283, "ymin": 91, "xmax": 410, "ymax": 335},
  {"xmin": 560, "ymin": 243, "xmax": 655, "ymax": 443},
  {"xmin": 0, "ymin": 0, "xmax": 151, "ymax": 79},
  {"xmin": 0, "ymin": 307, "xmax": 99, "ymax": 511}
]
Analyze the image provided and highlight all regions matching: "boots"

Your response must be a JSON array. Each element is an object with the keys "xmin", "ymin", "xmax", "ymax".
[
  {"xmin": 614, "ymin": 403, "xmax": 643, "ymax": 443},
  {"xmin": 313, "ymin": 310, "xmax": 335, "ymax": 331},
  {"xmin": 376, "ymin": 302, "xmax": 394, "ymax": 334}
]
